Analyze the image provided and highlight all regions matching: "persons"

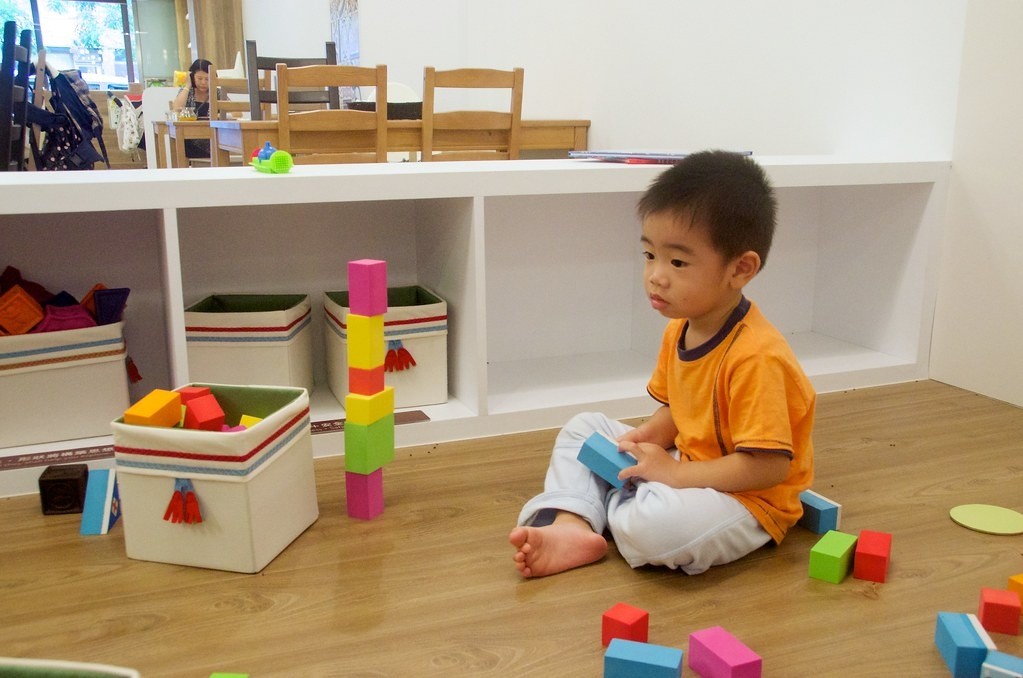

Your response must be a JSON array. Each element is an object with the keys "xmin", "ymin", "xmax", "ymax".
[
  {"xmin": 507, "ymin": 149, "xmax": 816, "ymax": 579},
  {"xmin": 173, "ymin": 59, "xmax": 230, "ymax": 157}
]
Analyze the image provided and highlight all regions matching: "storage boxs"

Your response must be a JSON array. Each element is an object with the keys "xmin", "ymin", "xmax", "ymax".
[
  {"xmin": 184, "ymin": 291, "xmax": 317, "ymax": 399},
  {"xmin": 109, "ymin": 381, "xmax": 319, "ymax": 575},
  {"xmin": 325, "ymin": 283, "xmax": 447, "ymax": 412},
  {"xmin": 0, "ymin": 319, "xmax": 131, "ymax": 449}
]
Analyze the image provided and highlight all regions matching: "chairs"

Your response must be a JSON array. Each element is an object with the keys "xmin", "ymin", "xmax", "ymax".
[
  {"xmin": 245, "ymin": 41, "xmax": 338, "ymax": 120},
  {"xmin": 206, "ymin": 65, "xmax": 272, "ymax": 167},
  {"xmin": 274, "ymin": 65, "xmax": 389, "ymax": 167},
  {"xmin": 0, "ymin": 21, "xmax": 52, "ymax": 172},
  {"xmin": 420, "ymin": 66, "xmax": 527, "ymax": 161}
]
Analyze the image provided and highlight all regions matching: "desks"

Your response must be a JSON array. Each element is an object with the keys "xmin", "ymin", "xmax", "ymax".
[
  {"xmin": 153, "ymin": 118, "xmax": 235, "ymax": 167},
  {"xmin": 209, "ymin": 118, "xmax": 591, "ymax": 169}
]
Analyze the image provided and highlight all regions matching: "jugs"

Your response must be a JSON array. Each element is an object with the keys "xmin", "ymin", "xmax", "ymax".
[{"xmin": 178, "ymin": 107, "xmax": 197, "ymax": 121}]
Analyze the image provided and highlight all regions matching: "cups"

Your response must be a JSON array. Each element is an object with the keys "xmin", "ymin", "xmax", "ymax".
[{"xmin": 167, "ymin": 111, "xmax": 177, "ymax": 121}]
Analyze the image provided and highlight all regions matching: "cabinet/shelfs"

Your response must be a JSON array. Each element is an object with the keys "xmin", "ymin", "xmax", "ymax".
[{"xmin": 0, "ymin": 158, "xmax": 938, "ymax": 499}]
